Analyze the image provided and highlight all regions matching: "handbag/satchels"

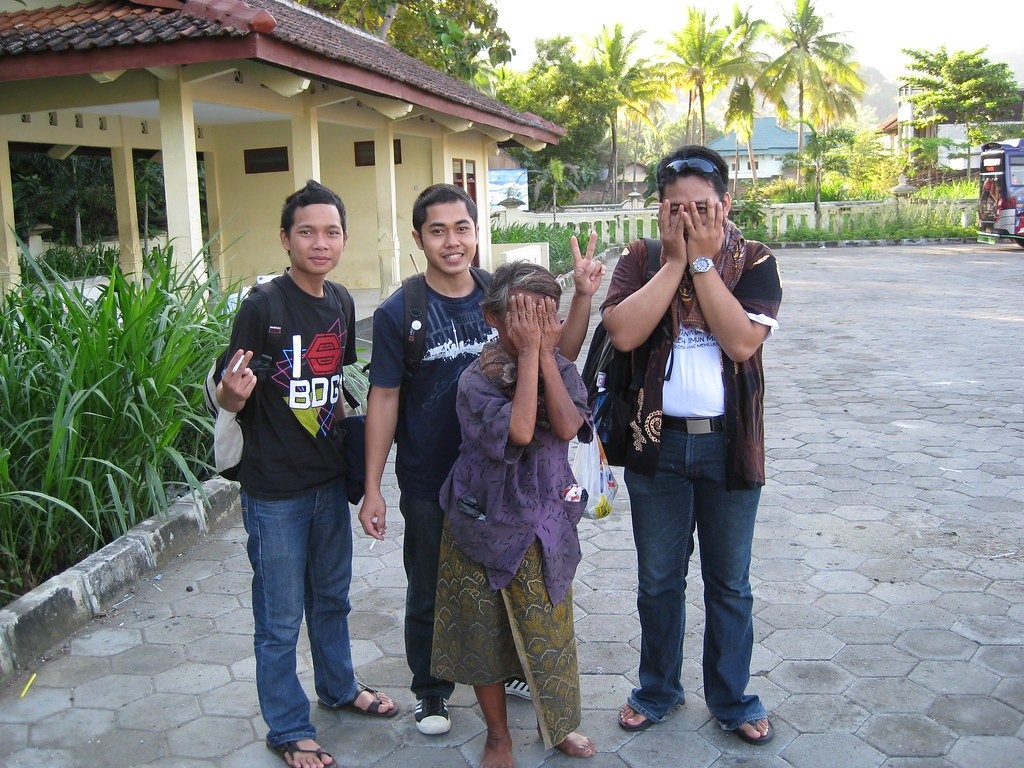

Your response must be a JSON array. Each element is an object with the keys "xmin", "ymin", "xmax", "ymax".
[
  {"xmin": 575, "ymin": 238, "xmax": 663, "ymax": 468},
  {"xmin": 565, "ymin": 421, "xmax": 619, "ymax": 520}
]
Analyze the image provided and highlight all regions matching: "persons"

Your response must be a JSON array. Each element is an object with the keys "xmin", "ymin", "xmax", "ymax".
[
  {"xmin": 358, "ymin": 183, "xmax": 606, "ymax": 734},
  {"xmin": 599, "ymin": 145, "xmax": 782, "ymax": 746},
  {"xmin": 213, "ymin": 179, "xmax": 398, "ymax": 768},
  {"xmin": 979, "ymin": 176, "xmax": 997, "ymax": 208},
  {"xmin": 430, "ymin": 262, "xmax": 588, "ymax": 767}
]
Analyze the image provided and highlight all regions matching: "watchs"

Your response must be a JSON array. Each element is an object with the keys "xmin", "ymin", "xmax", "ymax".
[{"xmin": 689, "ymin": 257, "xmax": 715, "ymax": 277}]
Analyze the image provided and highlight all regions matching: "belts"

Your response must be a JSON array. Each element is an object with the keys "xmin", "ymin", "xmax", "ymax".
[{"xmin": 661, "ymin": 412, "xmax": 724, "ymax": 435}]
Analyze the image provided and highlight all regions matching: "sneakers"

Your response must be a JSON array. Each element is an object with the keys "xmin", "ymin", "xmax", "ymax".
[
  {"xmin": 415, "ymin": 695, "xmax": 451, "ymax": 734},
  {"xmin": 505, "ymin": 678, "xmax": 533, "ymax": 699}
]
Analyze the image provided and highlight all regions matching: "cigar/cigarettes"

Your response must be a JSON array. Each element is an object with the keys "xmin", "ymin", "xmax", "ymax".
[
  {"xmin": 232, "ymin": 355, "xmax": 245, "ymax": 373},
  {"xmin": 369, "ymin": 532, "xmax": 382, "ymax": 551}
]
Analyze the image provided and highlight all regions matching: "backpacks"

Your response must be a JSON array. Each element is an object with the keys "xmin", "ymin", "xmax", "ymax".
[{"xmin": 204, "ymin": 283, "xmax": 351, "ymax": 481}]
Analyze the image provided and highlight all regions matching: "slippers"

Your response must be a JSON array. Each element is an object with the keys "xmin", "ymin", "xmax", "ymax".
[
  {"xmin": 317, "ymin": 681, "xmax": 398, "ymax": 717},
  {"xmin": 266, "ymin": 737, "xmax": 336, "ymax": 768},
  {"xmin": 733, "ymin": 716, "xmax": 774, "ymax": 746},
  {"xmin": 619, "ymin": 705, "xmax": 654, "ymax": 731}
]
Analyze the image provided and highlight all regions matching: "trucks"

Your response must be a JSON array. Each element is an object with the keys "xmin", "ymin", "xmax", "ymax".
[{"xmin": 974, "ymin": 138, "xmax": 1024, "ymax": 247}]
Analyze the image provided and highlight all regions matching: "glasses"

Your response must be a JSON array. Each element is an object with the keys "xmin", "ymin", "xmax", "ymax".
[{"xmin": 658, "ymin": 157, "xmax": 724, "ymax": 188}]
